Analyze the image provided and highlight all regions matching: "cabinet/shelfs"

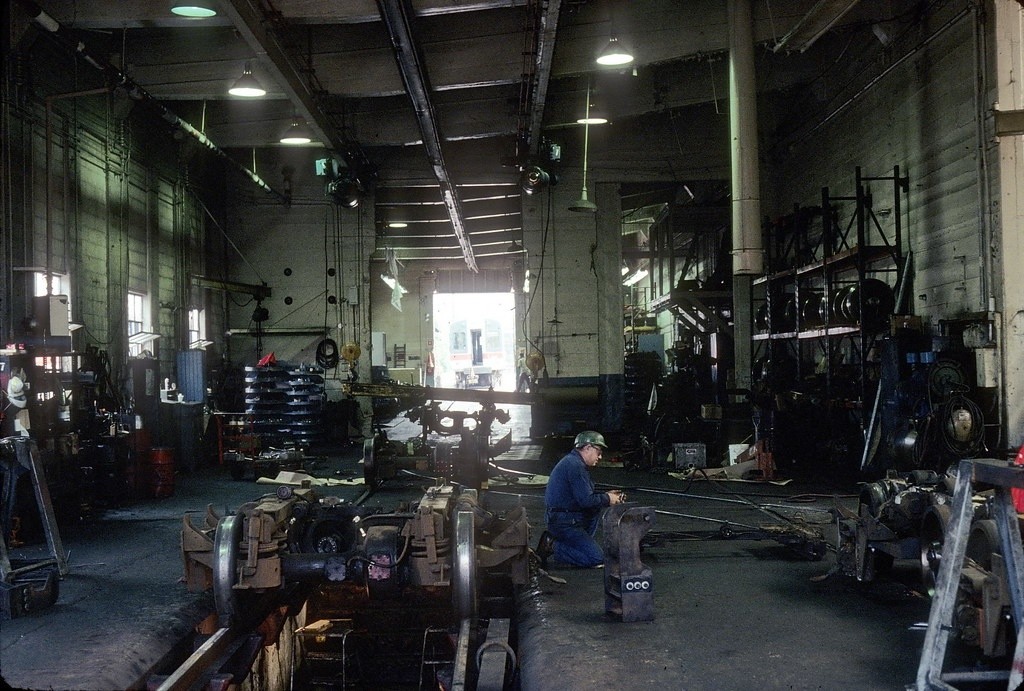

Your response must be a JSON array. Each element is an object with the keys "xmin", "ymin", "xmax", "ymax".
[
  {"xmin": 796, "ymin": 163, "xmax": 914, "ymax": 405},
  {"xmin": 751, "ymin": 265, "xmax": 799, "ymax": 380}
]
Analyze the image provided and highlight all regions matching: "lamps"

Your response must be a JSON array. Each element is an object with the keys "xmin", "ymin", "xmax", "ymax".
[
  {"xmin": 566, "ymin": 85, "xmax": 598, "ymax": 214},
  {"xmin": 228, "ymin": 61, "xmax": 266, "ymax": 98},
  {"xmin": 279, "ymin": 102, "xmax": 311, "ymax": 145},
  {"xmin": 576, "ymin": 76, "xmax": 607, "ymax": 124},
  {"xmin": 595, "ymin": 0, "xmax": 634, "ymax": 66},
  {"xmin": 170, "ymin": 0, "xmax": 217, "ymax": 19}
]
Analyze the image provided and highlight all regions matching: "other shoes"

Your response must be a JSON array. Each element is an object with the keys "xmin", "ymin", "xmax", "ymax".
[{"xmin": 536, "ymin": 530, "xmax": 557, "ymax": 561}]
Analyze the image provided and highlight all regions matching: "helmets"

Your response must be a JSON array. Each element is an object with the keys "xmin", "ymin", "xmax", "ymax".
[{"xmin": 574, "ymin": 431, "xmax": 608, "ymax": 449}]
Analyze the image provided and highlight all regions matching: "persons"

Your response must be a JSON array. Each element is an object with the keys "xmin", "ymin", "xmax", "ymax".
[{"xmin": 536, "ymin": 431, "xmax": 627, "ymax": 569}]
